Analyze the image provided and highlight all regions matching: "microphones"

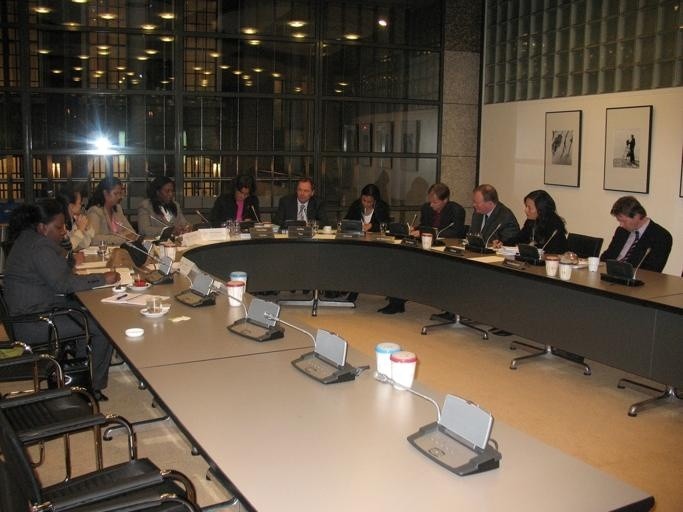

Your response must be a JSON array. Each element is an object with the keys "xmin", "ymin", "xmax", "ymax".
[
  {"xmin": 414, "ymin": 222, "xmax": 455, "ymax": 247},
  {"xmin": 384, "ymin": 213, "xmax": 417, "ymax": 240},
  {"xmin": 600, "ymin": 247, "xmax": 651, "ymax": 287},
  {"xmin": 209, "ymin": 284, "xmax": 286, "ymax": 342},
  {"xmin": 239, "ymin": 205, "xmax": 260, "ymax": 233},
  {"xmin": 153, "ymin": 256, "xmax": 216, "ymax": 308},
  {"xmin": 150, "ymin": 215, "xmax": 175, "ymax": 241},
  {"xmin": 114, "ymin": 220, "xmax": 145, "ymax": 243},
  {"xmin": 125, "ymin": 242, "xmax": 174, "ymax": 285},
  {"xmin": 515, "ymin": 229, "xmax": 558, "ymax": 266},
  {"xmin": 104, "ymin": 232, "xmax": 153, "ymax": 254},
  {"xmin": 465, "ymin": 223, "xmax": 502, "ymax": 254},
  {"xmin": 372, "ymin": 372, "xmax": 502, "ymax": 477},
  {"xmin": 195, "ymin": 209, "xmax": 212, "ymax": 231},
  {"xmin": 263, "ymin": 312, "xmax": 357, "ymax": 385}
]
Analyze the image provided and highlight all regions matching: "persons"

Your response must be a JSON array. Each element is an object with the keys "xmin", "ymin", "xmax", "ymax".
[
  {"xmin": 493, "ymin": 189, "xmax": 570, "ymax": 255},
  {"xmin": 430, "ymin": 183, "xmax": 520, "ymax": 323},
  {"xmin": 623, "ymin": 133, "xmax": 636, "ymax": 162},
  {"xmin": 324, "ymin": 184, "xmax": 390, "ymax": 300},
  {"xmin": 600, "ymin": 196, "xmax": 673, "ymax": 273},
  {"xmin": 3, "ymin": 177, "xmax": 139, "ymax": 402},
  {"xmin": 376, "ymin": 183, "xmax": 466, "ymax": 314},
  {"xmin": 210, "ymin": 175, "xmax": 260, "ymax": 227},
  {"xmin": 137, "ymin": 176, "xmax": 193, "ymax": 236},
  {"xmin": 271, "ymin": 177, "xmax": 320, "ymax": 296}
]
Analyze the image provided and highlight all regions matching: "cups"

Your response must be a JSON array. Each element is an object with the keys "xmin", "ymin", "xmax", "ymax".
[
  {"xmin": 230, "ymin": 270, "xmax": 247, "ymax": 294},
  {"xmin": 390, "ymin": 352, "xmax": 417, "ymax": 390},
  {"xmin": 147, "ymin": 296, "xmax": 161, "ymax": 312},
  {"xmin": 134, "ymin": 272, "xmax": 146, "ymax": 286},
  {"xmin": 559, "ymin": 261, "xmax": 573, "ymax": 281},
  {"xmin": 376, "ymin": 342, "xmax": 400, "ymax": 375},
  {"xmin": 588, "ymin": 257, "xmax": 600, "ymax": 272},
  {"xmin": 165, "ymin": 243, "xmax": 177, "ymax": 262},
  {"xmin": 336, "ymin": 220, "xmax": 342, "ymax": 232},
  {"xmin": 545, "ymin": 256, "xmax": 559, "ymax": 277},
  {"xmin": 226, "ymin": 280, "xmax": 245, "ymax": 308},
  {"xmin": 307, "ymin": 220, "xmax": 318, "ymax": 238},
  {"xmin": 421, "ymin": 233, "xmax": 432, "ymax": 250},
  {"xmin": 221, "ymin": 219, "xmax": 241, "ymax": 237}
]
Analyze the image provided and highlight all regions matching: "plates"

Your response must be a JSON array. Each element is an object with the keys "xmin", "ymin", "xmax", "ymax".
[
  {"xmin": 128, "ymin": 283, "xmax": 153, "ymax": 290},
  {"xmin": 124, "ymin": 327, "xmax": 144, "ymax": 338},
  {"xmin": 140, "ymin": 306, "xmax": 169, "ymax": 317}
]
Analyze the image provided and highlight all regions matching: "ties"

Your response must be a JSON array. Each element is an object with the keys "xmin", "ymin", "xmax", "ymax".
[
  {"xmin": 297, "ymin": 205, "xmax": 307, "ymax": 222},
  {"xmin": 618, "ymin": 231, "xmax": 639, "ymax": 263}
]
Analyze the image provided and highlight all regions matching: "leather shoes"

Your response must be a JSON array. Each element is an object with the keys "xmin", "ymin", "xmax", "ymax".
[
  {"xmin": 347, "ymin": 291, "xmax": 359, "ymax": 302},
  {"xmin": 489, "ymin": 328, "xmax": 513, "ymax": 336},
  {"xmin": 376, "ymin": 304, "xmax": 405, "ymax": 313},
  {"xmin": 429, "ymin": 312, "xmax": 456, "ymax": 323},
  {"xmin": 86, "ymin": 390, "xmax": 109, "ymax": 402}
]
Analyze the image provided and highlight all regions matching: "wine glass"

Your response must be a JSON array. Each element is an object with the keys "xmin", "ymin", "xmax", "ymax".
[
  {"xmin": 100, "ymin": 239, "xmax": 108, "ymax": 261},
  {"xmin": 380, "ymin": 223, "xmax": 387, "ymax": 236}
]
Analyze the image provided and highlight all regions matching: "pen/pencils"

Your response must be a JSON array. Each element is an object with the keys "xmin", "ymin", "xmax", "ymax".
[
  {"xmin": 319, "ymin": 232, "xmax": 335, "ymax": 235},
  {"xmin": 117, "ymin": 294, "xmax": 127, "ymax": 300}
]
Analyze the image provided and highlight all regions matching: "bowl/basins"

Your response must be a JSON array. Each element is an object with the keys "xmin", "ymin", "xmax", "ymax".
[{"xmin": 560, "ymin": 251, "xmax": 579, "ymax": 264}]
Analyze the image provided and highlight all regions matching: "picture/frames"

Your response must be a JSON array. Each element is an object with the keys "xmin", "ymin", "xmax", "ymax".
[
  {"xmin": 543, "ymin": 110, "xmax": 581, "ymax": 187},
  {"xmin": 603, "ymin": 104, "xmax": 653, "ymax": 194},
  {"xmin": 343, "ymin": 121, "xmax": 420, "ymax": 172}
]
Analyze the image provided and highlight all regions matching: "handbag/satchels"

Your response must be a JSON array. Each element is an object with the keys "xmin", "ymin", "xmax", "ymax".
[
  {"xmin": 47, "ymin": 357, "xmax": 92, "ymax": 388},
  {"xmin": 106, "ymin": 248, "xmax": 149, "ymax": 275}
]
